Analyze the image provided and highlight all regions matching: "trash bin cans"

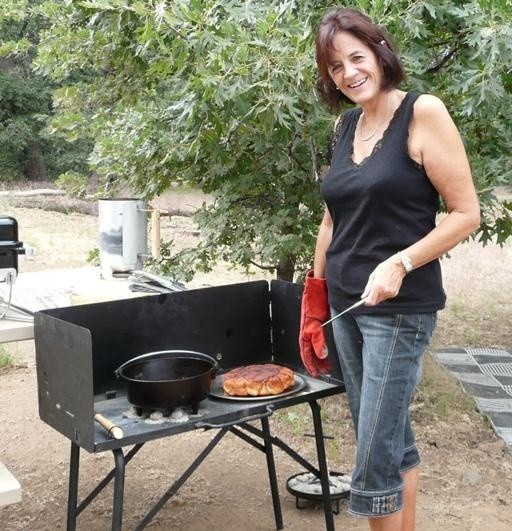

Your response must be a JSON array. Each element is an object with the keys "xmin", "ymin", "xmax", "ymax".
[{"xmin": 98, "ymin": 197, "xmax": 160, "ymax": 278}]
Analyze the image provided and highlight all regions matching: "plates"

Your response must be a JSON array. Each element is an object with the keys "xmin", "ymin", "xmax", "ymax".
[{"xmin": 211, "ymin": 374, "xmax": 306, "ymax": 401}]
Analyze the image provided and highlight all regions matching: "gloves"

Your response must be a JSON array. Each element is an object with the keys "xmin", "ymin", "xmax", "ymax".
[{"xmin": 296, "ymin": 267, "xmax": 328, "ymax": 377}]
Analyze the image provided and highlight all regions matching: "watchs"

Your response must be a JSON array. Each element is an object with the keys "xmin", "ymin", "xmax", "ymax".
[{"xmin": 396, "ymin": 249, "xmax": 414, "ymax": 273}]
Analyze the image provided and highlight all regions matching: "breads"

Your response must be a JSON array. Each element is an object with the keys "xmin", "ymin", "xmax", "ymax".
[{"xmin": 222, "ymin": 364, "xmax": 295, "ymax": 396}]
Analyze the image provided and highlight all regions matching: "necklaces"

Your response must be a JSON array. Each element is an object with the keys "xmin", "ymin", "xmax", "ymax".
[{"xmin": 350, "ymin": 97, "xmax": 395, "ymax": 142}]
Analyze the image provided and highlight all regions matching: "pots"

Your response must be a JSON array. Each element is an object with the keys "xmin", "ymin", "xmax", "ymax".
[{"xmin": 115, "ymin": 349, "xmax": 221, "ymax": 419}]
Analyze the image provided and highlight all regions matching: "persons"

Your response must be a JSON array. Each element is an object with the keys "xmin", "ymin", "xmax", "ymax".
[{"xmin": 299, "ymin": 8, "xmax": 481, "ymax": 531}]
